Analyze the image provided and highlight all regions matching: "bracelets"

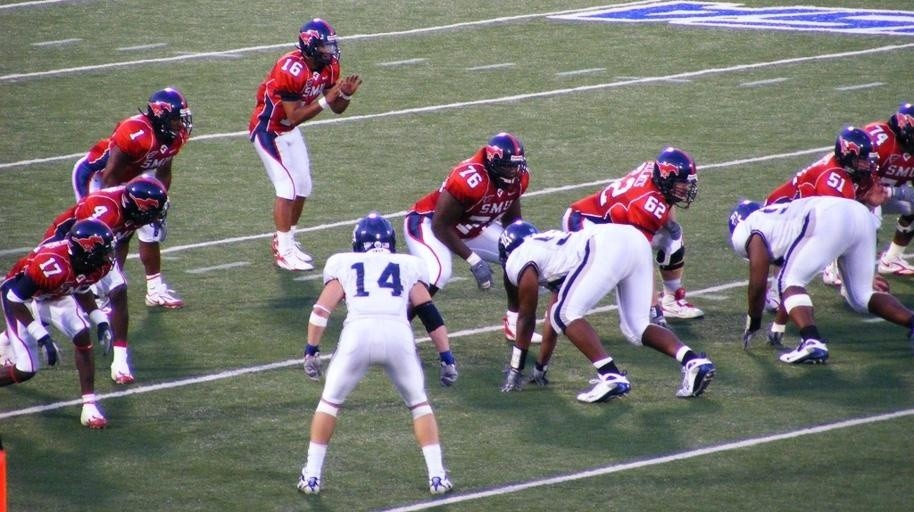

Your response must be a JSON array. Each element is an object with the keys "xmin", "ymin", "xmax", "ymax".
[
  {"xmin": 650, "ymin": 305, "xmax": 663, "ymax": 320},
  {"xmin": 318, "ymin": 98, "xmax": 329, "ymax": 110},
  {"xmin": 339, "ymin": 89, "xmax": 352, "ymax": 101},
  {"xmin": 509, "ymin": 346, "xmax": 528, "ymax": 370},
  {"xmin": 89, "ymin": 310, "xmax": 109, "ymax": 326},
  {"xmin": 466, "ymin": 251, "xmax": 481, "ymax": 266},
  {"xmin": 437, "ymin": 349, "xmax": 454, "ymax": 365},
  {"xmin": 27, "ymin": 320, "xmax": 48, "ymax": 341},
  {"xmin": 746, "ymin": 312, "xmax": 761, "ymax": 331},
  {"xmin": 304, "ymin": 344, "xmax": 320, "ymax": 357}
]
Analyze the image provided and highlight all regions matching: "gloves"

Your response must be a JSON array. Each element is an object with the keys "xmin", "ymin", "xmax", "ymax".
[
  {"xmin": 439, "ymin": 351, "xmax": 458, "ymax": 387},
  {"xmin": 766, "ymin": 321, "xmax": 786, "ymax": 345},
  {"xmin": 742, "ymin": 308, "xmax": 761, "ymax": 350},
  {"xmin": 524, "ymin": 362, "xmax": 550, "ymax": 386},
  {"xmin": 502, "ymin": 367, "xmax": 523, "ymax": 392},
  {"xmin": 97, "ymin": 322, "xmax": 112, "ymax": 355},
  {"xmin": 303, "ymin": 344, "xmax": 324, "ymax": 382},
  {"xmin": 650, "ymin": 305, "xmax": 672, "ymax": 329},
  {"xmin": 36, "ymin": 334, "xmax": 62, "ymax": 368},
  {"xmin": 153, "ymin": 221, "xmax": 166, "ymax": 242},
  {"xmin": 470, "ymin": 258, "xmax": 494, "ymax": 290}
]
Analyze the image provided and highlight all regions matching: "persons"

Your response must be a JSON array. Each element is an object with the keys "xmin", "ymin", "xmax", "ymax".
[
  {"xmin": 249, "ymin": 18, "xmax": 362, "ymax": 272},
  {"xmin": 407, "ymin": 132, "xmax": 543, "ymax": 345},
  {"xmin": 0, "ymin": 219, "xmax": 117, "ymax": 427},
  {"xmin": 764, "ymin": 103, "xmax": 914, "ymax": 313},
  {"xmin": 296, "ymin": 213, "xmax": 458, "ymax": 497},
  {"xmin": 729, "ymin": 196, "xmax": 913, "ymax": 364},
  {"xmin": 6, "ymin": 174, "xmax": 171, "ymax": 386},
  {"xmin": 71, "ymin": 87, "xmax": 192, "ymax": 310},
  {"xmin": 497, "ymin": 220, "xmax": 716, "ymax": 405},
  {"xmin": 563, "ymin": 147, "xmax": 704, "ymax": 329}
]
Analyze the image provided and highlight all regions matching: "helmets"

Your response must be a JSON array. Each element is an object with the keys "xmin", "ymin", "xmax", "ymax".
[
  {"xmin": 121, "ymin": 173, "xmax": 168, "ymax": 227},
  {"xmin": 834, "ymin": 127, "xmax": 881, "ymax": 179},
  {"xmin": 64, "ymin": 217, "xmax": 115, "ymax": 276},
  {"xmin": 729, "ymin": 200, "xmax": 764, "ymax": 233},
  {"xmin": 886, "ymin": 103, "xmax": 913, "ymax": 151},
  {"xmin": 351, "ymin": 212, "xmax": 395, "ymax": 254},
  {"xmin": 298, "ymin": 18, "xmax": 340, "ymax": 67},
  {"xmin": 486, "ymin": 131, "xmax": 526, "ymax": 192},
  {"xmin": 147, "ymin": 88, "xmax": 192, "ymax": 142},
  {"xmin": 652, "ymin": 147, "xmax": 698, "ymax": 208},
  {"xmin": 498, "ymin": 219, "xmax": 538, "ymax": 262}
]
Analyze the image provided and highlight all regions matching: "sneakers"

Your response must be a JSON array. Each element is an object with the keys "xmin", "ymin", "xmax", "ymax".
[
  {"xmin": 297, "ymin": 467, "xmax": 321, "ymax": 495},
  {"xmin": 823, "ymin": 264, "xmax": 842, "ymax": 286},
  {"xmin": 676, "ymin": 352, "xmax": 716, "ymax": 397},
  {"xmin": 110, "ymin": 363, "xmax": 135, "ymax": 384},
  {"xmin": 765, "ymin": 288, "xmax": 782, "ymax": 311},
  {"xmin": 272, "ymin": 237, "xmax": 312, "ymax": 272},
  {"xmin": 879, "ymin": 250, "xmax": 914, "ymax": 275},
  {"xmin": 577, "ymin": 367, "xmax": 632, "ymax": 403},
  {"xmin": 657, "ymin": 287, "xmax": 705, "ymax": 319},
  {"xmin": 503, "ymin": 316, "xmax": 543, "ymax": 344},
  {"xmin": 428, "ymin": 469, "xmax": 454, "ymax": 495},
  {"xmin": 780, "ymin": 338, "xmax": 828, "ymax": 364},
  {"xmin": 145, "ymin": 283, "xmax": 183, "ymax": 309},
  {"xmin": 81, "ymin": 403, "xmax": 106, "ymax": 429}
]
